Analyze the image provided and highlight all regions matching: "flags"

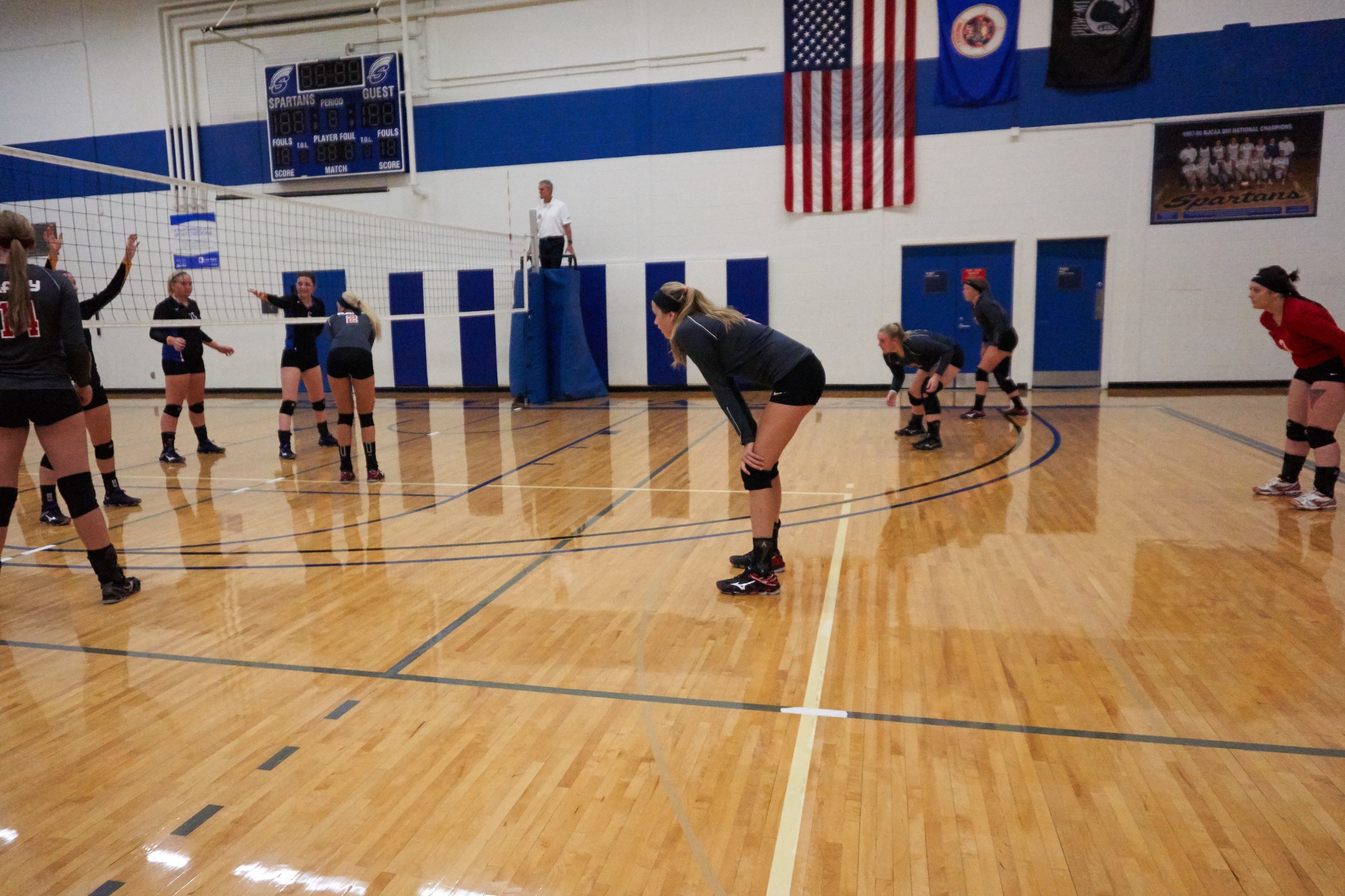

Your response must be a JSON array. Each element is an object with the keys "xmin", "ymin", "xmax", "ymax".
[
  {"xmin": 931, "ymin": 0, "xmax": 1021, "ymax": 106},
  {"xmin": 783, "ymin": 0, "xmax": 917, "ymax": 212},
  {"xmin": 1045, "ymin": 0, "xmax": 1155, "ymax": 93}
]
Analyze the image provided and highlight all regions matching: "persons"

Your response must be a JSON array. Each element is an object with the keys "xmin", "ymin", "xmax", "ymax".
[
  {"xmin": 877, "ymin": 322, "xmax": 965, "ymax": 450},
  {"xmin": 323, "ymin": 292, "xmax": 384, "ymax": 484},
  {"xmin": 959, "ymin": 277, "xmax": 1029, "ymax": 420},
  {"xmin": 1177, "ymin": 134, "xmax": 1296, "ymax": 195},
  {"xmin": 0, "ymin": 210, "xmax": 142, "ymax": 604},
  {"xmin": 652, "ymin": 282, "xmax": 826, "ymax": 595},
  {"xmin": 247, "ymin": 271, "xmax": 339, "ymax": 459},
  {"xmin": 1248, "ymin": 265, "xmax": 1345, "ymax": 511},
  {"xmin": 149, "ymin": 269, "xmax": 234, "ymax": 463},
  {"xmin": 526, "ymin": 180, "xmax": 575, "ymax": 269}
]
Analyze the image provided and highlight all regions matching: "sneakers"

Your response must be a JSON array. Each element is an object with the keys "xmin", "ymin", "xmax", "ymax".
[
  {"xmin": 101, "ymin": 576, "xmax": 141, "ymax": 604},
  {"xmin": 366, "ymin": 465, "xmax": 385, "ymax": 482},
  {"xmin": 1289, "ymin": 487, "xmax": 1337, "ymax": 511},
  {"xmin": 40, "ymin": 507, "xmax": 71, "ymax": 525},
  {"xmin": 278, "ymin": 447, "xmax": 297, "ymax": 460},
  {"xmin": 103, "ymin": 490, "xmax": 142, "ymax": 506},
  {"xmin": 894, "ymin": 419, "xmax": 925, "ymax": 437},
  {"xmin": 1254, "ymin": 493, "xmax": 1294, "ymax": 513},
  {"xmin": 1005, "ymin": 407, "xmax": 1029, "ymax": 417},
  {"xmin": 1251, "ymin": 473, "xmax": 1302, "ymax": 495},
  {"xmin": 317, "ymin": 434, "xmax": 338, "ymax": 447},
  {"xmin": 716, "ymin": 566, "xmax": 781, "ymax": 596},
  {"xmin": 340, "ymin": 468, "xmax": 356, "ymax": 484},
  {"xmin": 196, "ymin": 441, "xmax": 226, "ymax": 454},
  {"xmin": 958, "ymin": 408, "xmax": 987, "ymax": 419},
  {"xmin": 1287, "ymin": 508, "xmax": 1336, "ymax": 524},
  {"xmin": 158, "ymin": 448, "xmax": 187, "ymax": 463},
  {"xmin": 716, "ymin": 594, "xmax": 783, "ymax": 620},
  {"xmin": 729, "ymin": 552, "xmax": 787, "ymax": 573},
  {"xmin": 911, "ymin": 431, "xmax": 942, "ymax": 451}
]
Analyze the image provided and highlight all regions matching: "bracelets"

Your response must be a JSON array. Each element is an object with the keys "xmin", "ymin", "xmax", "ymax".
[{"xmin": 568, "ymin": 240, "xmax": 572, "ymax": 243}]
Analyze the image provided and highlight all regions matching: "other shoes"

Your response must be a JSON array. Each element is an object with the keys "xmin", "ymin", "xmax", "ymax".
[
  {"xmin": 160, "ymin": 461, "xmax": 187, "ymax": 473},
  {"xmin": 197, "ymin": 452, "xmax": 226, "ymax": 464},
  {"xmin": 104, "ymin": 505, "xmax": 143, "ymax": 518}
]
